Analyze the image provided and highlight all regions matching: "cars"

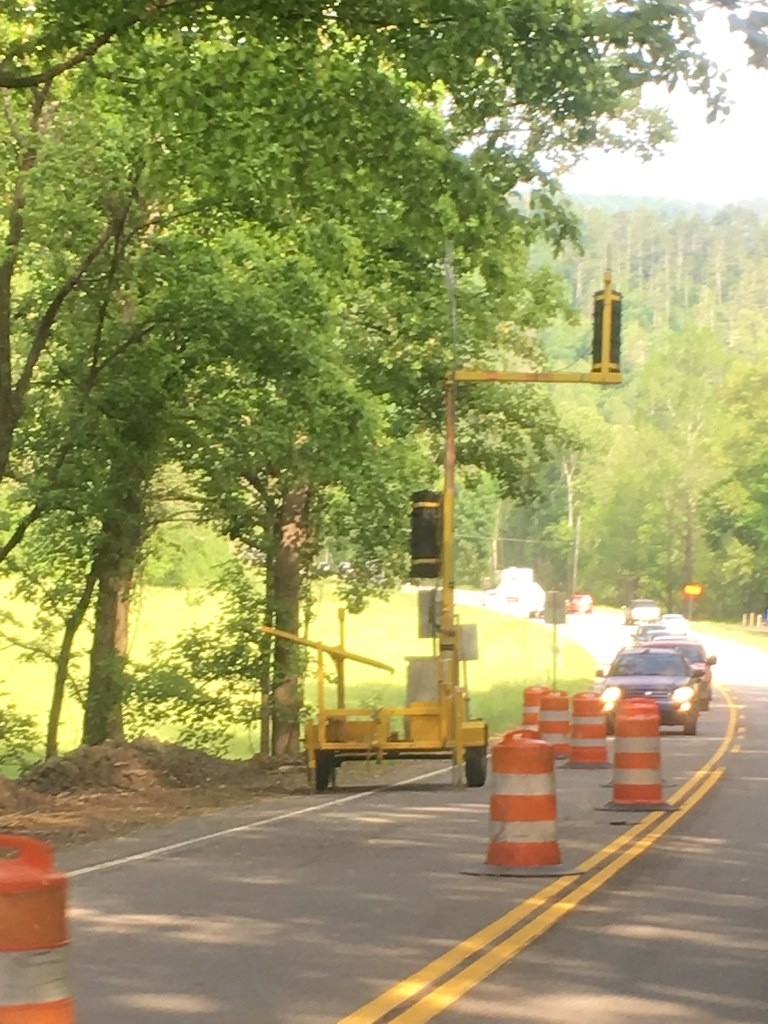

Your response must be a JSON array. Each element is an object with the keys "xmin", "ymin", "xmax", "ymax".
[{"xmin": 556, "ymin": 579, "xmax": 717, "ymax": 733}]
